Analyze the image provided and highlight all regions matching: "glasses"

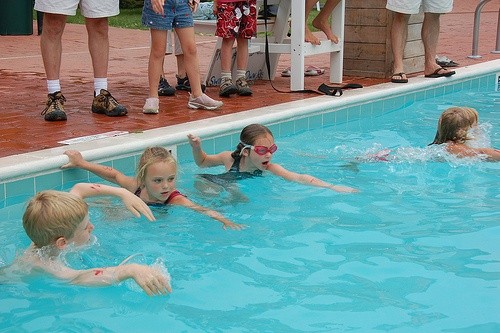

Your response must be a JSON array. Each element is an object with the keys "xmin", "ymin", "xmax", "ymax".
[{"xmin": 254, "ymin": 144, "xmax": 277, "ymax": 156}]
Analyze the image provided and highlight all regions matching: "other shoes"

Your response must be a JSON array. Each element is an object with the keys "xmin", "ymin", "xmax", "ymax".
[{"xmin": 436, "ymin": 55, "xmax": 459, "ymax": 66}]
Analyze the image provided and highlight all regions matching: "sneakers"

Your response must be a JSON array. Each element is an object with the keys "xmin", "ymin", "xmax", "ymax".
[
  {"xmin": 175, "ymin": 74, "xmax": 205, "ymax": 92},
  {"xmin": 91, "ymin": 89, "xmax": 128, "ymax": 117},
  {"xmin": 41, "ymin": 92, "xmax": 68, "ymax": 120},
  {"xmin": 188, "ymin": 91, "xmax": 223, "ymax": 111},
  {"xmin": 219, "ymin": 78, "xmax": 237, "ymax": 96},
  {"xmin": 143, "ymin": 97, "xmax": 159, "ymax": 114},
  {"xmin": 156, "ymin": 76, "xmax": 175, "ymax": 95},
  {"xmin": 236, "ymin": 77, "xmax": 252, "ymax": 95}
]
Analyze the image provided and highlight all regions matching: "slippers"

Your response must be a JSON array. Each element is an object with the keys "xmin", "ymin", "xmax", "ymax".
[
  {"xmin": 392, "ymin": 73, "xmax": 408, "ymax": 83},
  {"xmin": 304, "ymin": 64, "xmax": 326, "ymax": 76},
  {"xmin": 281, "ymin": 67, "xmax": 290, "ymax": 77},
  {"xmin": 424, "ymin": 66, "xmax": 455, "ymax": 77}
]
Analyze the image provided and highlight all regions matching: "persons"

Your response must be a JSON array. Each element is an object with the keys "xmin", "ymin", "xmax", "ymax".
[
  {"xmin": 21, "ymin": 180, "xmax": 173, "ymax": 298},
  {"xmin": 188, "ymin": 124, "xmax": 322, "ymax": 184},
  {"xmin": 141, "ymin": 0, "xmax": 224, "ymax": 114},
  {"xmin": 61, "ymin": 147, "xmax": 192, "ymax": 207},
  {"xmin": 33, "ymin": 0, "xmax": 128, "ymax": 121},
  {"xmin": 158, "ymin": 29, "xmax": 207, "ymax": 95},
  {"xmin": 289, "ymin": 0, "xmax": 341, "ymax": 45},
  {"xmin": 384, "ymin": 0, "xmax": 456, "ymax": 84},
  {"xmin": 433, "ymin": 107, "xmax": 500, "ymax": 161},
  {"xmin": 213, "ymin": 0, "xmax": 258, "ymax": 96}
]
